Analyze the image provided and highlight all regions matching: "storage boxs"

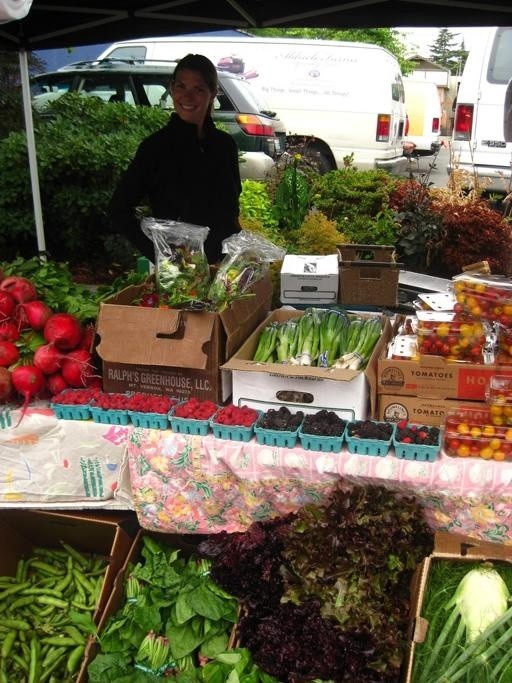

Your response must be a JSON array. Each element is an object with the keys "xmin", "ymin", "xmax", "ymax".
[
  {"xmin": 219, "ymin": 308, "xmax": 392, "ymax": 423},
  {"xmin": 272, "ymin": 260, "xmax": 511, "ymax": 428},
  {"xmin": 280, "ymin": 254, "xmax": 339, "ymax": 304},
  {"xmin": 76, "ymin": 529, "xmax": 249, "ymax": 683},
  {"xmin": 336, "ymin": 243, "xmax": 404, "ymax": 307},
  {"xmin": 402, "ymin": 530, "xmax": 512, "ymax": 683},
  {"xmin": 136, "ymin": 256, "xmax": 155, "ymax": 275},
  {"xmin": 94, "ymin": 265, "xmax": 273, "ymax": 407},
  {"xmin": 1, "ymin": 508, "xmax": 133, "ymax": 683}
]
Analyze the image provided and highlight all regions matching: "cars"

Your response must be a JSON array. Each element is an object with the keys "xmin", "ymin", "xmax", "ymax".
[{"xmin": 23, "ymin": 55, "xmax": 293, "ymax": 170}]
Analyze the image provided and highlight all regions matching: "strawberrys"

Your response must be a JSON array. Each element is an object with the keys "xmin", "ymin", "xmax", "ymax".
[{"xmin": 50, "ymin": 382, "xmax": 257, "ymax": 427}]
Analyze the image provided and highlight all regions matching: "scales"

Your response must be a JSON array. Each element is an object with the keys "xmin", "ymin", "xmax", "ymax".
[{"xmin": 397, "ymin": 269, "xmax": 452, "ymax": 311}]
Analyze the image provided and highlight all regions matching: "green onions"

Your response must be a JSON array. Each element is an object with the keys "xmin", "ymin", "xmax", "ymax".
[{"xmin": 252, "ymin": 306, "xmax": 382, "ymax": 371}]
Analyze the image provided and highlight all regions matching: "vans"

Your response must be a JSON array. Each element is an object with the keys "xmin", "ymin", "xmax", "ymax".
[
  {"xmin": 90, "ymin": 35, "xmax": 409, "ymax": 190},
  {"xmin": 403, "ymin": 73, "xmax": 444, "ymax": 155},
  {"xmin": 446, "ymin": 27, "xmax": 511, "ymax": 212}
]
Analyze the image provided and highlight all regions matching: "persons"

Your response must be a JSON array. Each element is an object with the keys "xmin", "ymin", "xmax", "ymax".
[{"xmin": 110, "ymin": 52, "xmax": 244, "ymax": 265}]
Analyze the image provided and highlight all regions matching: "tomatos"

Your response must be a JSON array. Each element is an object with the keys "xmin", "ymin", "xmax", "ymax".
[{"xmin": 421, "ymin": 270, "xmax": 512, "ymax": 463}]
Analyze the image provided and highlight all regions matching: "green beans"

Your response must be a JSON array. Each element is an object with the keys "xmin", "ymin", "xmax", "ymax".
[{"xmin": 0, "ymin": 539, "xmax": 110, "ymax": 683}]
